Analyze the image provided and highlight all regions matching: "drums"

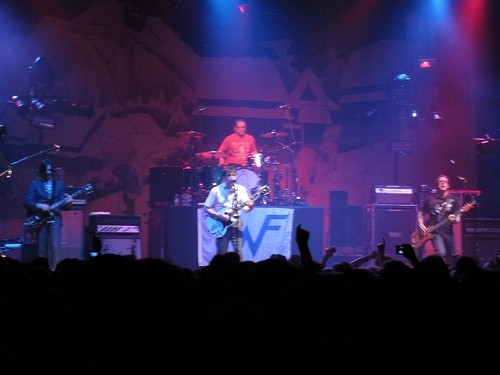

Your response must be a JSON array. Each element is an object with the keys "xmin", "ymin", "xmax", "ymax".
[
  {"xmin": 189, "ymin": 164, "xmax": 217, "ymax": 194},
  {"xmin": 219, "ymin": 168, "xmax": 264, "ymax": 204},
  {"xmin": 246, "ymin": 153, "xmax": 269, "ymax": 168}
]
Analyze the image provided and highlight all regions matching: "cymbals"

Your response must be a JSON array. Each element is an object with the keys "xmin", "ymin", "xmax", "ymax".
[
  {"xmin": 258, "ymin": 131, "xmax": 290, "ymax": 138},
  {"xmin": 196, "ymin": 151, "xmax": 229, "ymax": 159},
  {"xmin": 177, "ymin": 131, "xmax": 207, "ymax": 138}
]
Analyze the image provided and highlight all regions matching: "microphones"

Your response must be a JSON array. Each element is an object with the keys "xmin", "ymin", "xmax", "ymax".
[
  {"xmin": 277, "ymin": 105, "xmax": 290, "ymax": 111},
  {"xmin": 472, "ymin": 137, "xmax": 489, "ymax": 141},
  {"xmin": 199, "ymin": 107, "xmax": 207, "ymax": 111},
  {"xmin": 231, "ymin": 179, "xmax": 237, "ymax": 183}
]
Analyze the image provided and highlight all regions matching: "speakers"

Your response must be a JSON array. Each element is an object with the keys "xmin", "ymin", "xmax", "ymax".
[
  {"xmin": 366, "ymin": 204, "xmax": 418, "ymax": 269},
  {"xmin": 462, "ymin": 217, "xmax": 500, "ymax": 267},
  {"xmin": 89, "ymin": 233, "xmax": 143, "ymax": 261},
  {"xmin": 328, "ymin": 191, "xmax": 364, "ymax": 249},
  {"xmin": 148, "ymin": 166, "xmax": 176, "ymax": 207},
  {"xmin": 48, "ymin": 208, "xmax": 87, "ymax": 271},
  {"xmin": 148, "ymin": 206, "xmax": 198, "ymax": 271}
]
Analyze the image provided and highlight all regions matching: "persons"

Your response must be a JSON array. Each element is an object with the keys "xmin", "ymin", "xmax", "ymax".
[
  {"xmin": 24, "ymin": 160, "xmax": 72, "ymax": 269},
  {"xmin": 218, "ymin": 119, "xmax": 262, "ymax": 199},
  {"xmin": 0, "ymin": 223, "xmax": 500, "ymax": 375},
  {"xmin": 204, "ymin": 169, "xmax": 254, "ymax": 260},
  {"xmin": 417, "ymin": 174, "xmax": 462, "ymax": 264}
]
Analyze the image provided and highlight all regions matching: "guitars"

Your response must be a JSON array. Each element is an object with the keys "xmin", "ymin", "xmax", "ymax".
[
  {"xmin": 205, "ymin": 185, "xmax": 272, "ymax": 239},
  {"xmin": 19, "ymin": 183, "xmax": 95, "ymax": 235},
  {"xmin": 409, "ymin": 201, "xmax": 476, "ymax": 249}
]
